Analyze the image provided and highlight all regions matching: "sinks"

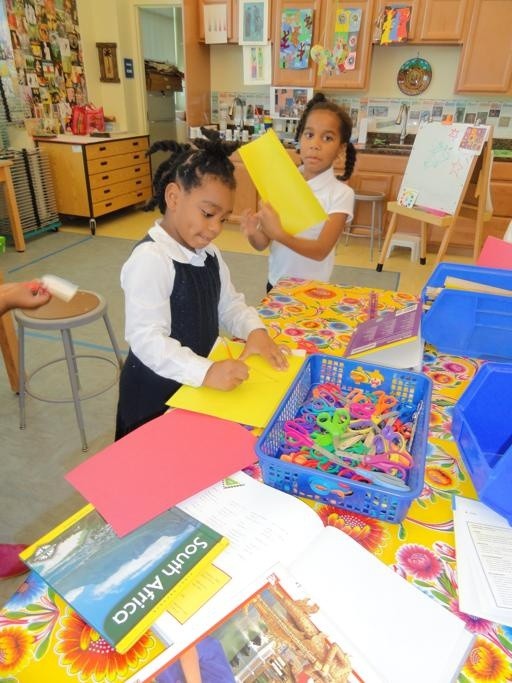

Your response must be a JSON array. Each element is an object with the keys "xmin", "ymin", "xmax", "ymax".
[{"xmin": 370, "ymin": 142, "xmax": 413, "ymax": 152}]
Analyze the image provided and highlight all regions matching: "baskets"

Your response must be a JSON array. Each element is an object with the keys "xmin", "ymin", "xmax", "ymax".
[{"xmin": 255, "ymin": 353, "xmax": 433, "ymax": 525}]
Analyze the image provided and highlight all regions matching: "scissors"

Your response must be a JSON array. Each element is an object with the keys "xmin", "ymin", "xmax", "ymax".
[{"xmin": 279, "ymin": 382, "xmax": 424, "ymax": 518}]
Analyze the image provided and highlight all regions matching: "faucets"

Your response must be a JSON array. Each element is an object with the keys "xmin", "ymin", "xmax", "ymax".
[
  {"xmin": 394, "ymin": 103, "xmax": 410, "ymax": 144},
  {"xmin": 227, "ymin": 96, "xmax": 244, "ymax": 129}
]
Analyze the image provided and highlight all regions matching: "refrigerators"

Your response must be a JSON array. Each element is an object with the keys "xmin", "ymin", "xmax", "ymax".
[{"xmin": 148, "ymin": 91, "xmax": 177, "ymax": 197}]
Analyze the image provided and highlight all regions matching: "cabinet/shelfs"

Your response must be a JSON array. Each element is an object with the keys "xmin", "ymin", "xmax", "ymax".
[
  {"xmin": 37, "ymin": 136, "xmax": 154, "ymax": 235},
  {"xmin": 454, "ymin": 0, "xmax": 512, "ymax": 94},
  {"xmin": 430, "ymin": 162, "xmax": 512, "ymax": 249},
  {"xmin": 222, "ymin": 148, "xmax": 264, "ymax": 225},
  {"xmin": 332, "ymin": 152, "xmax": 394, "ymax": 239},
  {"xmin": 373, "ymin": 0, "xmax": 472, "ymax": 46},
  {"xmin": 271, "ymin": 0, "xmax": 373, "ymax": 92},
  {"xmin": 198, "ymin": 0, "xmax": 271, "ymax": 45}
]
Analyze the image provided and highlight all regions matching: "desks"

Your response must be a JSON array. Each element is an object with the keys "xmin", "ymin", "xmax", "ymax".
[
  {"xmin": 0, "ymin": 274, "xmax": 511, "ymax": 682},
  {"xmin": 0, "ymin": 159, "xmax": 27, "ymax": 252}
]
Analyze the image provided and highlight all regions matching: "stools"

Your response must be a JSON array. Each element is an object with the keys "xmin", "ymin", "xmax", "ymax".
[
  {"xmin": 14, "ymin": 288, "xmax": 124, "ymax": 452},
  {"xmin": 333, "ymin": 188, "xmax": 386, "ymax": 263},
  {"xmin": 385, "ymin": 233, "xmax": 423, "ymax": 264}
]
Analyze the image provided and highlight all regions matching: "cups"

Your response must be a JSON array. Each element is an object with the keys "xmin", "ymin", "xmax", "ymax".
[
  {"xmin": 241, "ymin": 130, "xmax": 249, "ymax": 143},
  {"xmin": 233, "ymin": 129, "xmax": 241, "ymax": 142},
  {"xmin": 218, "ymin": 131, "xmax": 225, "ymax": 139},
  {"xmin": 226, "ymin": 129, "xmax": 233, "ymax": 141}
]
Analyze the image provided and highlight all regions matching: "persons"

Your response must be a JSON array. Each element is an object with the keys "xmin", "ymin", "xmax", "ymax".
[
  {"xmin": 242, "ymin": 95, "xmax": 356, "ymax": 294},
  {"xmin": 1, "ymin": 280, "xmax": 52, "ymax": 579},
  {"xmin": 115, "ymin": 131, "xmax": 292, "ymax": 443}
]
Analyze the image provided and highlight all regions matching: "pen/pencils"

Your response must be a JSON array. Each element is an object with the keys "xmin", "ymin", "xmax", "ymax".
[{"xmin": 222, "ymin": 336, "xmax": 233, "ymax": 360}]
